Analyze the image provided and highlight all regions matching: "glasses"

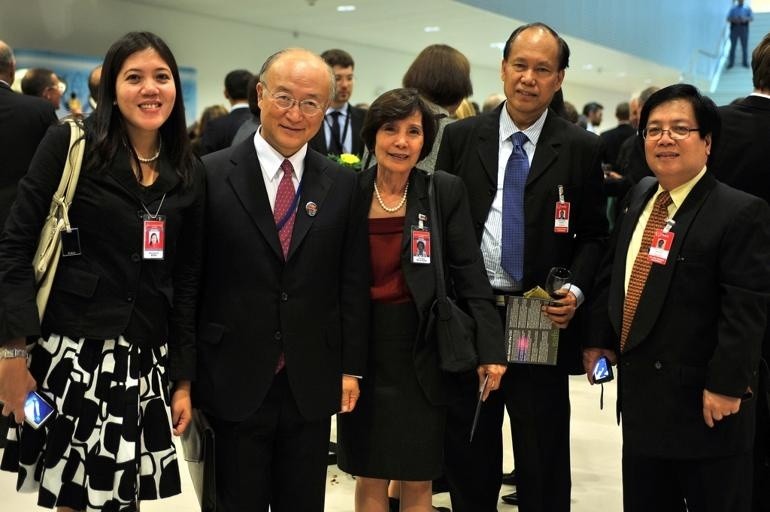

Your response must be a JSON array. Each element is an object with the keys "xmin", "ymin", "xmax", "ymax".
[
  {"xmin": 262, "ymin": 79, "xmax": 330, "ymax": 119},
  {"xmin": 640, "ymin": 122, "xmax": 707, "ymax": 140}
]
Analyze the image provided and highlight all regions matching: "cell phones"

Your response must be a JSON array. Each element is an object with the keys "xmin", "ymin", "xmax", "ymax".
[
  {"xmin": 591, "ymin": 355, "xmax": 614, "ymax": 383},
  {"xmin": 25, "ymin": 391, "xmax": 54, "ymax": 429}
]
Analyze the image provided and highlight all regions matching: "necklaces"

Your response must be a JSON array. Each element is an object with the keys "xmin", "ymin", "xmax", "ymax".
[
  {"xmin": 372, "ymin": 180, "xmax": 409, "ymax": 211},
  {"xmin": 122, "ymin": 134, "xmax": 162, "ymax": 163}
]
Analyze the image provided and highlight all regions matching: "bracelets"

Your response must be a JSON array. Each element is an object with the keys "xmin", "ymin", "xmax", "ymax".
[{"xmin": 0, "ymin": 348, "xmax": 32, "ymax": 368}]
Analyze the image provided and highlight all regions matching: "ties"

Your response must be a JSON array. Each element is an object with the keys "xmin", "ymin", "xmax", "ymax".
[
  {"xmin": 499, "ymin": 133, "xmax": 531, "ymax": 282},
  {"xmin": 273, "ymin": 161, "xmax": 300, "ymax": 254},
  {"xmin": 621, "ymin": 194, "xmax": 672, "ymax": 353}
]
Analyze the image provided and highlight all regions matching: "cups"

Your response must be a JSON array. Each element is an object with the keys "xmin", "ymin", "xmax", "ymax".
[{"xmin": 546, "ymin": 264, "xmax": 572, "ymax": 300}]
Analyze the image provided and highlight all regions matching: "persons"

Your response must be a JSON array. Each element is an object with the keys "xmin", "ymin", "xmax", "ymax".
[
  {"xmin": 558, "ymin": 210, "xmax": 565, "ymax": 219},
  {"xmin": 727, "ymin": 0, "xmax": 752, "ymax": 70},
  {"xmin": 657, "ymin": 239, "xmax": 665, "ymax": 248},
  {"xmin": 195, "ymin": 47, "xmax": 366, "ymax": 510},
  {"xmin": 148, "ymin": 233, "xmax": 160, "ymax": 246},
  {"xmin": 435, "ymin": 21, "xmax": 609, "ymax": 512},
  {"xmin": 332, "ymin": 85, "xmax": 509, "ymax": 512},
  {"xmin": 417, "ymin": 239, "xmax": 427, "ymax": 257},
  {"xmin": 578, "ymin": 83, "xmax": 770, "ymax": 512},
  {"xmin": 1, "ymin": 28, "xmax": 194, "ymax": 512}
]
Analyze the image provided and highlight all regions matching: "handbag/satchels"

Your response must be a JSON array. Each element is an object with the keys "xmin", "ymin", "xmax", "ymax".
[
  {"xmin": 178, "ymin": 407, "xmax": 224, "ymax": 511},
  {"xmin": 32, "ymin": 189, "xmax": 76, "ymax": 326},
  {"xmin": 432, "ymin": 291, "xmax": 479, "ymax": 377}
]
